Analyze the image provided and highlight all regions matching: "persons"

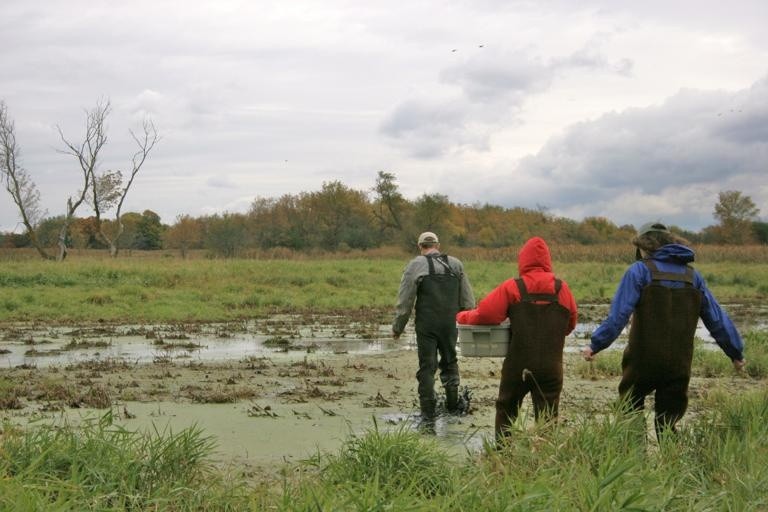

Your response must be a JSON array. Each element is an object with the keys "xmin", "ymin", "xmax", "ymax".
[
  {"xmin": 456, "ymin": 237, "xmax": 577, "ymax": 449},
  {"xmin": 583, "ymin": 222, "xmax": 747, "ymax": 443},
  {"xmin": 392, "ymin": 231, "xmax": 474, "ymax": 436}
]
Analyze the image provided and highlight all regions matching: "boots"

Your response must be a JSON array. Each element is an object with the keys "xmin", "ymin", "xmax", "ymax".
[
  {"xmin": 420, "ymin": 399, "xmax": 436, "ymax": 420},
  {"xmin": 445, "ymin": 387, "xmax": 458, "ymax": 410}
]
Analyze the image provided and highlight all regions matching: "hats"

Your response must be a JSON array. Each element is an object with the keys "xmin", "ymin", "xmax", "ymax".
[
  {"xmin": 418, "ymin": 231, "xmax": 439, "ymax": 245},
  {"xmin": 635, "ymin": 221, "xmax": 671, "ymax": 261}
]
